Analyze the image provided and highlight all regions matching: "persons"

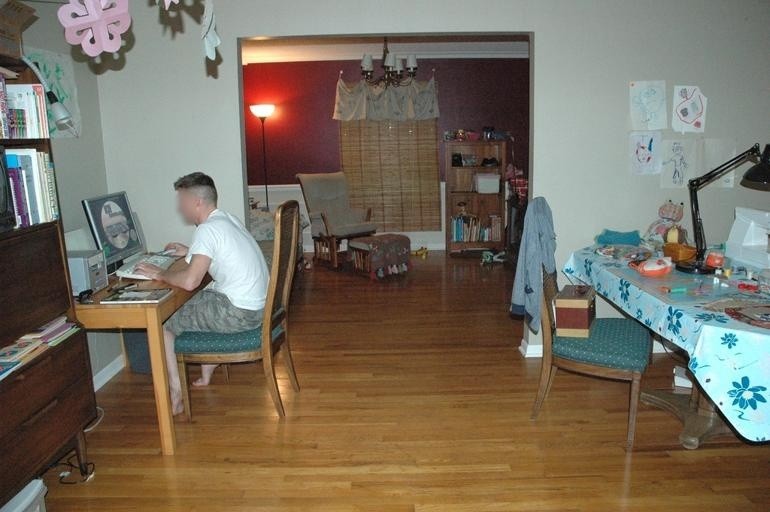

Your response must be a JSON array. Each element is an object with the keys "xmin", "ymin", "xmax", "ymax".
[{"xmin": 133, "ymin": 172, "xmax": 271, "ymax": 415}]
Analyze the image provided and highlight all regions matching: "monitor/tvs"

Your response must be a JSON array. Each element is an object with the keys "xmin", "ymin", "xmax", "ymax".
[
  {"xmin": 0, "ymin": 145, "xmax": 17, "ymax": 234},
  {"xmin": 81, "ymin": 191, "xmax": 145, "ymax": 277}
]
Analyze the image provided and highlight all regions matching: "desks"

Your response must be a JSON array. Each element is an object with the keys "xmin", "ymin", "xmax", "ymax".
[
  {"xmin": 72, "ymin": 253, "xmax": 215, "ymax": 456},
  {"xmin": 564, "ymin": 246, "xmax": 769, "ymax": 448},
  {"xmin": 249, "ymin": 203, "xmax": 303, "ymax": 273}
]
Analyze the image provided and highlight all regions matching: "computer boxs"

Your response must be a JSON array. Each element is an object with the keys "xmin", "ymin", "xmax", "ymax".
[{"xmin": 122, "ymin": 323, "xmax": 152, "ymax": 372}]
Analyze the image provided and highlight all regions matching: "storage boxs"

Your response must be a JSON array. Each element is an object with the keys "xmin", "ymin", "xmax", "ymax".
[
  {"xmin": 551, "ymin": 282, "xmax": 597, "ymax": 341},
  {"xmin": 472, "ymin": 172, "xmax": 500, "ymax": 195}
]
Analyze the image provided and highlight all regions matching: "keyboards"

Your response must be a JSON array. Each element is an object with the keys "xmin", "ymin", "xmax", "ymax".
[{"xmin": 116, "ymin": 254, "xmax": 183, "ymax": 280}]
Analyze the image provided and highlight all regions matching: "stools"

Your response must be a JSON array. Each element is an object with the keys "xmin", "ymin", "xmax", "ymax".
[{"xmin": 348, "ymin": 233, "xmax": 411, "ymax": 280}]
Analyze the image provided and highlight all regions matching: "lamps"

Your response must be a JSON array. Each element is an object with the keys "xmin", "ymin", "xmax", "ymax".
[
  {"xmin": 18, "ymin": 57, "xmax": 71, "ymax": 125},
  {"xmin": 679, "ymin": 141, "xmax": 769, "ymax": 273},
  {"xmin": 248, "ymin": 104, "xmax": 279, "ymax": 211},
  {"xmin": 361, "ymin": 35, "xmax": 417, "ymax": 88}
]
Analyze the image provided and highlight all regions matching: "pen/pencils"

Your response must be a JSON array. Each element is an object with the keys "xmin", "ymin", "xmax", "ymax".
[{"xmin": 108, "ymin": 283, "xmax": 138, "ymax": 293}]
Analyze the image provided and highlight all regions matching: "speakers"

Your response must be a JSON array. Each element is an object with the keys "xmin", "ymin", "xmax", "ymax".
[{"xmin": 66, "ymin": 250, "xmax": 109, "ymax": 296}]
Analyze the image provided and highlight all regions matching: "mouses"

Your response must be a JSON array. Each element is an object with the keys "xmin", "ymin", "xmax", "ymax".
[{"xmin": 162, "ymin": 248, "xmax": 177, "ymax": 255}]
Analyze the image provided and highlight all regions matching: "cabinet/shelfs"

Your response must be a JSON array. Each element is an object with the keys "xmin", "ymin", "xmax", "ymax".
[
  {"xmin": 443, "ymin": 138, "xmax": 509, "ymax": 256},
  {"xmin": 0, "ymin": 61, "xmax": 76, "ymax": 357},
  {"xmin": 2, "ymin": 329, "xmax": 97, "ymax": 509}
]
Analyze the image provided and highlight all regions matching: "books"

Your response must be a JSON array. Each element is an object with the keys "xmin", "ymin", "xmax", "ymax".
[
  {"xmin": 99, "ymin": 286, "xmax": 174, "ymax": 306},
  {"xmin": 451, "ymin": 213, "xmax": 503, "ymax": 241},
  {"xmin": 0, "ymin": 316, "xmax": 80, "ymax": 379},
  {"xmin": 0, "ymin": 78, "xmax": 59, "ymax": 228}
]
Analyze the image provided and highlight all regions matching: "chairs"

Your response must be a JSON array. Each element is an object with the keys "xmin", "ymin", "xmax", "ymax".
[
  {"xmin": 295, "ymin": 170, "xmax": 378, "ymax": 270},
  {"xmin": 530, "ymin": 196, "xmax": 651, "ymax": 448},
  {"xmin": 174, "ymin": 199, "xmax": 302, "ymax": 419}
]
